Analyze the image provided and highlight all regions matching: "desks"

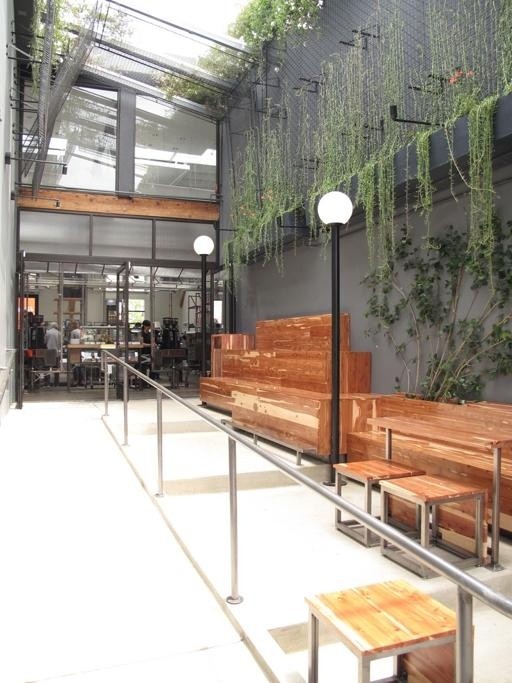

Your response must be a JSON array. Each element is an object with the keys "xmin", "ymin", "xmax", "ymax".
[{"xmin": 367, "ymin": 416, "xmax": 512, "ymax": 571}]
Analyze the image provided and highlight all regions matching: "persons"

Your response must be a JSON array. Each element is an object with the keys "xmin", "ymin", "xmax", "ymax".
[
  {"xmin": 69, "ymin": 321, "xmax": 81, "ymax": 387},
  {"xmin": 44, "ymin": 322, "xmax": 59, "ymax": 387},
  {"xmin": 141, "ymin": 320, "xmax": 157, "ymax": 388}
]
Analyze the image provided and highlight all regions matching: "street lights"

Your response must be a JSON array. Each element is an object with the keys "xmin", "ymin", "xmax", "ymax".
[
  {"xmin": 192, "ymin": 233, "xmax": 217, "ymax": 377},
  {"xmin": 313, "ymin": 188, "xmax": 355, "ymax": 490}
]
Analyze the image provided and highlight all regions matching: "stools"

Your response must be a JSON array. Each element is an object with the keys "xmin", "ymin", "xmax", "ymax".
[
  {"xmin": 305, "ymin": 579, "xmax": 475, "ymax": 683},
  {"xmin": 378, "ymin": 473, "xmax": 489, "ymax": 579},
  {"xmin": 332, "ymin": 458, "xmax": 426, "ymax": 548}
]
